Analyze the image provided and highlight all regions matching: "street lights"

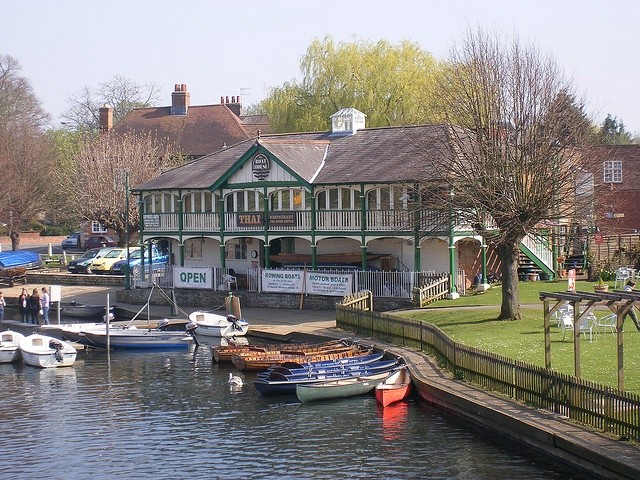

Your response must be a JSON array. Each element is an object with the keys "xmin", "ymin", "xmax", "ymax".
[{"xmin": 121, "ymin": 172, "xmax": 130, "ymax": 290}]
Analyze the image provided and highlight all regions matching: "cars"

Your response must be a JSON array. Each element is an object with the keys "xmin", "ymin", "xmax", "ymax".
[
  {"xmin": 90, "ymin": 248, "xmax": 148, "ymax": 275},
  {"xmin": 68, "ymin": 246, "xmax": 103, "ymax": 275},
  {"xmin": 85, "ymin": 235, "xmax": 116, "ymax": 251},
  {"xmin": 112, "ymin": 247, "xmax": 170, "ymax": 274},
  {"xmin": 62, "ymin": 231, "xmax": 82, "ymax": 249}
]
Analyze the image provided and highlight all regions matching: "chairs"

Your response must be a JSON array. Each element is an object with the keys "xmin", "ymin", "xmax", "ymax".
[
  {"xmin": 549, "ymin": 302, "xmax": 568, "ymax": 317},
  {"xmin": 560, "ymin": 316, "xmax": 578, "ymax": 343},
  {"xmin": 597, "ymin": 314, "xmax": 617, "ymax": 338},
  {"xmin": 615, "ymin": 267, "xmax": 637, "ymax": 287},
  {"xmin": 222, "ymin": 273, "xmax": 238, "ymax": 291},
  {"xmin": 579, "ymin": 316, "xmax": 596, "ymax": 343}
]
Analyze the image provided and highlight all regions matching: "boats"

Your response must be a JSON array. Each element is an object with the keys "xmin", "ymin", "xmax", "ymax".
[
  {"xmin": 267, "ymin": 352, "xmax": 384, "ymax": 371},
  {"xmin": 294, "ymin": 368, "xmax": 406, "ymax": 403},
  {"xmin": 253, "ymin": 366, "xmax": 397, "ymax": 397},
  {"xmin": 232, "ymin": 343, "xmax": 372, "ymax": 372},
  {"xmin": 188, "ymin": 311, "xmax": 249, "ymax": 338},
  {"xmin": 20, "ymin": 333, "xmax": 77, "ymax": 368},
  {"xmin": 211, "ymin": 336, "xmax": 360, "ymax": 363},
  {"xmin": 85, "ymin": 329, "xmax": 191, "ymax": 349},
  {"xmin": 259, "ymin": 358, "xmax": 399, "ymax": 381},
  {"xmin": 376, "ymin": 367, "xmax": 412, "ymax": 408},
  {"xmin": 0, "ymin": 330, "xmax": 26, "ymax": 364}
]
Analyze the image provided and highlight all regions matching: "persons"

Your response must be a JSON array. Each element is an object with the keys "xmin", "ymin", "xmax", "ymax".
[
  {"xmin": 0, "ymin": 292, "xmax": 6, "ymax": 320},
  {"xmin": 19, "ymin": 287, "xmax": 28, "ymax": 322},
  {"xmin": 30, "ymin": 288, "xmax": 41, "ymax": 325},
  {"xmin": 41, "ymin": 288, "xmax": 52, "ymax": 325},
  {"xmin": 623, "ymin": 280, "xmax": 640, "ymax": 332}
]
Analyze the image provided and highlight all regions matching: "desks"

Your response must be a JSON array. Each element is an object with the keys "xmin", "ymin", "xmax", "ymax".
[{"xmin": 560, "ymin": 300, "xmax": 619, "ymax": 334}]
